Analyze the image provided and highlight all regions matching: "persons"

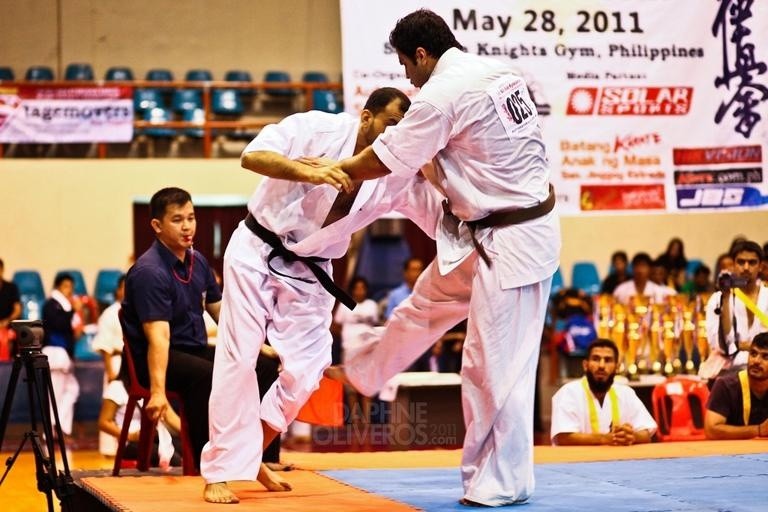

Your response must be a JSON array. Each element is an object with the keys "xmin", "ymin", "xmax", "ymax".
[
  {"xmin": 0, "ymin": 258, "xmax": 22, "ymax": 329},
  {"xmin": 333, "ymin": 274, "xmax": 380, "ymax": 326},
  {"xmin": 382, "ymin": 255, "xmax": 443, "ymax": 372},
  {"xmin": 549, "ymin": 337, "xmax": 659, "ymax": 446},
  {"xmin": 292, "ymin": 5, "xmax": 560, "ymax": 510},
  {"xmin": 600, "ymin": 234, "xmax": 746, "ymax": 303},
  {"xmin": 703, "ymin": 330, "xmax": 768, "ymax": 440},
  {"xmin": 198, "ymin": 86, "xmax": 447, "ymax": 504},
  {"xmin": 706, "ymin": 240, "xmax": 768, "ymax": 371},
  {"xmin": 96, "ymin": 186, "xmax": 295, "ymax": 473},
  {"xmin": 41, "ymin": 271, "xmax": 85, "ymax": 446}
]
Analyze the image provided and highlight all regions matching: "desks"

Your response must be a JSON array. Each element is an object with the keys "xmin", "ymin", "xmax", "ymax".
[{"xmin": 552, "ymin": 374, "xmax": 711, "ymax": 386}]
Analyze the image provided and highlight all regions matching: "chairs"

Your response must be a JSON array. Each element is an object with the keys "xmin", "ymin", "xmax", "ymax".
[
  {"xmin": 0, "ymin": 64, "xmax": 343, "ymax": 157},
  {"xmin": 545, "ymin": 300, "xmax": 565, "ymax": 330},
  {"xmin": 57, "ymin": 271, "xmax": 88, "ymax": 296},
  {"xmin": 652, "ymin": 378, "xmax": 711, "ymax": 442},
  {"xmin": 572, "ymin": 263, "xmax": 601, "ymax": 297},
  {"xmin": 550, "ymin": 267, "xmax": 565, "ymax": 297},
  {"xmin": 19, "ymin": 294, "xmax": 46, "ymax": 320},
  {"xmin": 74, "ymin": 333, "xmax": 104, "ymax": 361},
  {"xmin": 687, "ymin": 260, "xmax": 703, "ymax": 272},
  {"xmin": 556, "ymin": 310, "xmax": 596, "ymax": 358},
  {"xmin": 12, "ymin": 271, "xmax": 46, "ymax": 301},
  {"xmin": 94, "ymin": 270, "xmax": 123, "ymax": 304},
  {"xmin": 609, "ymin": 263, "xmax": 632, "ymax": 274},
  {"xmin": 113, "ymin": 308, "xmax": 200, "ymax": 475}
]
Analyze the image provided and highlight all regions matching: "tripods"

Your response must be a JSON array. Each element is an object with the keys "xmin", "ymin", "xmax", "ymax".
[{"xmin": 0, "ymin": 319, "xmax": 81, "ymax": 512}]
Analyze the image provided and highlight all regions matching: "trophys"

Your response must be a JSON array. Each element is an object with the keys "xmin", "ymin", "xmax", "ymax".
[{"xmin": 597, "ymin": 292, "xmax": 709, "ymax": 382}]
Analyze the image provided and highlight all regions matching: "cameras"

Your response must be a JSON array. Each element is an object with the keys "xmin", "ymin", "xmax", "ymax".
[{"xmin": 715, "ymin": 270, "xmax": 748, "ymax": 287}]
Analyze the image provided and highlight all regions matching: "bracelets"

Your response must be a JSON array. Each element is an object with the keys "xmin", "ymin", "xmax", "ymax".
[{"xmin": 757, "ymin": 424, "xmax": 762, "ymax": 436}]
